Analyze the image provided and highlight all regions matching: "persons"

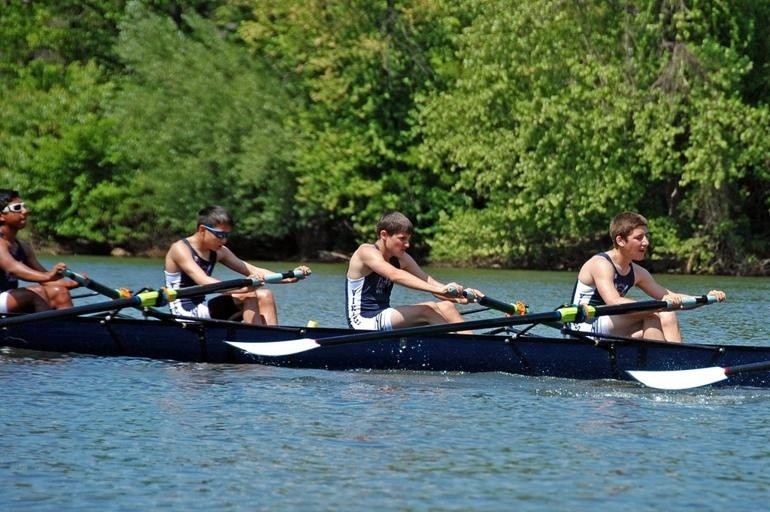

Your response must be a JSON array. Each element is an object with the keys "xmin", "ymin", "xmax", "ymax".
[
  {"xmin": 0, "ymin": 188, "xmax": 89, "ymax": 317},
  {"xmin": 345, "ymin": 209, "xmax": 485, "ymax": 335},
  {"xmin": 568, "ymin": 211, "xmax": 726, "ymax": 344},
  {"xmin": 163, "ymin": 204, "xmax": 310, "ymax": 326}
]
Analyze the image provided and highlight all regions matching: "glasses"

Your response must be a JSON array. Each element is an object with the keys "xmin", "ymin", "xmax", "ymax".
[
  {"xmin": 203, "ymin": 227, "xmax": 232, "ymax": 238},
  {"xmin": 2, "ymin": 201, "xmax": 28, "ymax": 213}
]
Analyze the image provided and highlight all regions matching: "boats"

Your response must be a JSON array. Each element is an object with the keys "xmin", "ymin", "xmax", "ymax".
[{"xmin": 0, "ymin": 313, "xmax": 766, "ymax": 388}]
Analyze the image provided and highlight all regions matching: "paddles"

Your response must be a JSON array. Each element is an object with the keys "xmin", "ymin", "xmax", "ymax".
[
  {"xmin": 223, "ymin": 295, "xmax": 716, "ymax": 356},
  {"xmin": 625, "ymin": 360, "xmax": 770, "ymax": 391}
]
